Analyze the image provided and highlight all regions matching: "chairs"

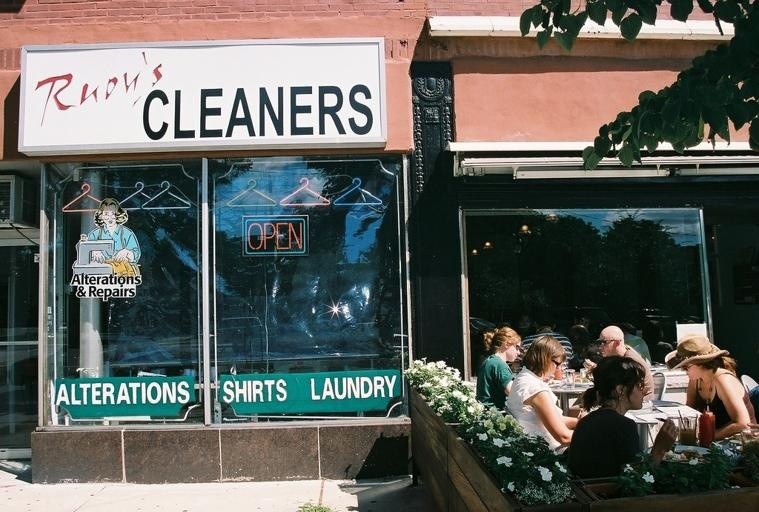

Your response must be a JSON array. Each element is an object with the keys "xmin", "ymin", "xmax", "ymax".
[{"xmin": 652, "ymin": 371, "xmax": 667, "ymax": 400}]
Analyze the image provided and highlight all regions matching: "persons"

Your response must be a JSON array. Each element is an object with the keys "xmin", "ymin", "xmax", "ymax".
[
  {"xmin": 663, "ymin": 334, "xmax": 759, "ymax": 443},
  {"xmin": 516, "ymin": 311, "xmax": 674, "ymax": 372},
  {"xmin": 80, "ymin": 197, "xmax": 142, "ymax": 264},
  {"xmin": 506, "ymin": 334, "xmax": 588, "ymax": 450},
  {"xmin": 562, "ymin": 354, "xmax": 679, "ymax": 479},
  {"xmin": 475, "ymin": 325, "xmax": 522, "ymax": 415},
  {"xmin": 582, "ymin": 325, "xmax": 655, "ymax": 404}
]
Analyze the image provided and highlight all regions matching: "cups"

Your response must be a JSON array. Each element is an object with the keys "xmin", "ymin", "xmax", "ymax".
[
  {"xmin": 698, "ymin": 415, "xmax": 716, "ymax": 448},
  {"xmin": 676, "ymin": 413, "xmax": 697, "ymax": 445},
  {"xmin": 565, "ymin": 368, "xmax": 575, "ymax": 389},
  {"xmin": 579, "ymin": 368, "xmax": 588, "ymax": 382}
]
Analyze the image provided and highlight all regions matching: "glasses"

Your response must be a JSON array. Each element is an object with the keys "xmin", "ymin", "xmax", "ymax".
[
  {"xmin": 677, "ymin": 365, "xmax": 695, "ymax": 371},
  {"xmin": 634, "ymin": 381, "xmax": 645, "ymax": 390},
  {"xmin": 592, "ymin": 337, "xmax": 622, "ymax": 345},
  {"xmin": 551, "ymin": 359, "xmax": 561, "ymax": 367},
  {"xmin": 511, "ymin": 343, "xmax": 520, "ymax": 351}
]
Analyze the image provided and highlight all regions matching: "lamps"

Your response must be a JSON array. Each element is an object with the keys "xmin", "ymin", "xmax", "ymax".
[{"xmin": 509, "ymin": 165, "xmax": 676, "ymax": 179}]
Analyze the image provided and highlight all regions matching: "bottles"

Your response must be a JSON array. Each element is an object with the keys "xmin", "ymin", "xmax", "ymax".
[{"xmin": 554, "ymin": 367, "xmax": 562, "ymax": 380}]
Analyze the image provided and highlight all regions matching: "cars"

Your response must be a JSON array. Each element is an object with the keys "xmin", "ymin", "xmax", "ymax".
[{"xmin": 68, "ymin": 315, "xmax": 381, "ymax": 365}]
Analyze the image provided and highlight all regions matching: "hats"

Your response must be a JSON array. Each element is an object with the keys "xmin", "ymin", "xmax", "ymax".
[{"xmin": 664, "ymin": 332, "xmax": 729, "ymax": 371}]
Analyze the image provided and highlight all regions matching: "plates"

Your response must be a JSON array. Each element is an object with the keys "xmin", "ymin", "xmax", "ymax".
[{"xmin": 574, "ymin": 383, "xmax": 589, "ymax": 387}]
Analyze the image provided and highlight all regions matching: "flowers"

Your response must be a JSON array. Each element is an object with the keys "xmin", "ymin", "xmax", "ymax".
[
  {"xmin": 403, "ymin": 356, "xmax": 485, "ymax": 424},
  {"xmin": 459, "ymin": 405, "xmax": 576, "ymax": 509},
  {"xmin": 618, "ymin": 441, "xmax": 757, "ymax": 497}
]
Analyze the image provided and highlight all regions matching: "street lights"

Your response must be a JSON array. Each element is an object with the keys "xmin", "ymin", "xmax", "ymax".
[{"xmin": 469, "ymin": 214, "xmax": 564, "ymax": 337}]
